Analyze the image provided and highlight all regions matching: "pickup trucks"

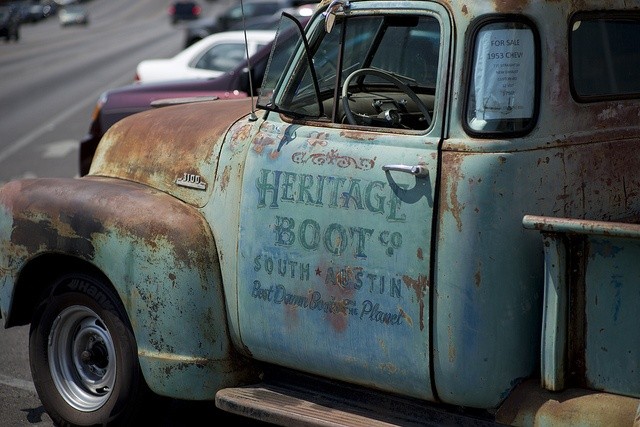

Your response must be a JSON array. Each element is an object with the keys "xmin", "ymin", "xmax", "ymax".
[{"xmin": 1, "ymin": 0, "xmax": 637, "ymax": 427}]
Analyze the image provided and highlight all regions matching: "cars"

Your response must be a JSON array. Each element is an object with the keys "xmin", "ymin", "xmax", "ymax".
[
  {"xmin": 134, "ymin": 29, "xmax": 282, "ymax": 83},
  {"xmin": 79, "ymin": 19, "xmax": 311, "ymax": 177}
]
